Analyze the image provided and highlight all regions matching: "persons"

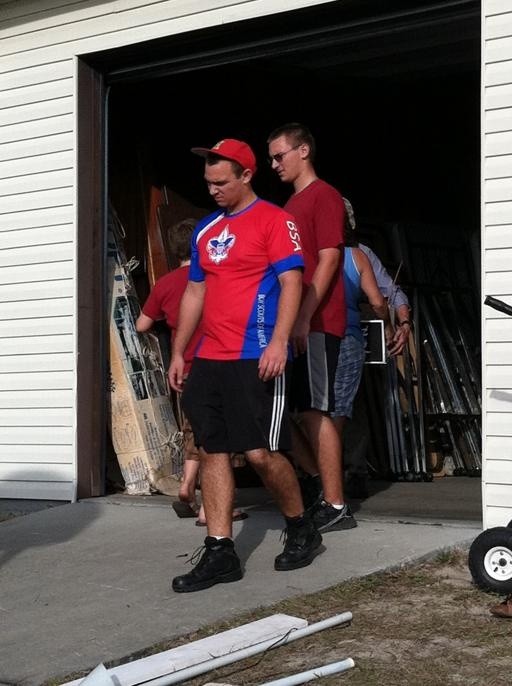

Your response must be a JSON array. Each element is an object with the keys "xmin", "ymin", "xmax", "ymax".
[
  {"xmin": 136, "ymin": 217, "xmax": 205, "ymax": 519},
  {"xmin": 266, "ymin": 125, "xmax": 349, "ymax": 532},
  {"xmin": 168, "ymin": 136, "xmax": 325, "ymax": 594},
  {"xmin": 323, "ymin": 243, "xmax": 395, "ymax": 501},
  {"xmin": 337, "ymin": 192, "xmax": 413, "ymax": 501}
]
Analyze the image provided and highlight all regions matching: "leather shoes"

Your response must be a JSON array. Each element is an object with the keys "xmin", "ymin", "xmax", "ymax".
[
  {"xmin": 173, "ymin": 536, "xmax": 243, "ymax": 592},
  {"xmin": 274, "ymin": 513, "xmax": 323, "ymax": 571}
]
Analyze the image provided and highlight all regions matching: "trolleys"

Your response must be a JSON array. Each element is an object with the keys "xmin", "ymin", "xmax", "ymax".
[{"xmin": 467, "ymin": 295, "xmax": 512, "ymax": 597}]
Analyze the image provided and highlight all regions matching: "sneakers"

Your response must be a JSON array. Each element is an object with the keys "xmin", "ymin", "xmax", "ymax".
[{"xmin": 292, "ymin": 473, "xmax": 357, "ymax": 532}]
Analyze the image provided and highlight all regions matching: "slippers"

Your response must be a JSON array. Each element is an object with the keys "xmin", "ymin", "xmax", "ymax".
[{"xmin": 172, "ymin": 498, "xmax": 247, "ymax": 527}]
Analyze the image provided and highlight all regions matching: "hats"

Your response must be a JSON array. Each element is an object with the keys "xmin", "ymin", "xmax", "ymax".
[{"xmin": 191, "ymin": 138, "xmax": 256, "ymax": 176}]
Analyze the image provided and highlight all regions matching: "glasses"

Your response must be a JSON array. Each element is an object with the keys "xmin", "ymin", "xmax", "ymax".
[{"xmin": 267, "ymin": 143, "xmax": 302, "ymax": 163}]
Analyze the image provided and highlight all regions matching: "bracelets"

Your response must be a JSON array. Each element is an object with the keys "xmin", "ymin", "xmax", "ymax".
[{"xmin": 399, "ymin": 319, "xmax": 411, "ymax": 326}]
